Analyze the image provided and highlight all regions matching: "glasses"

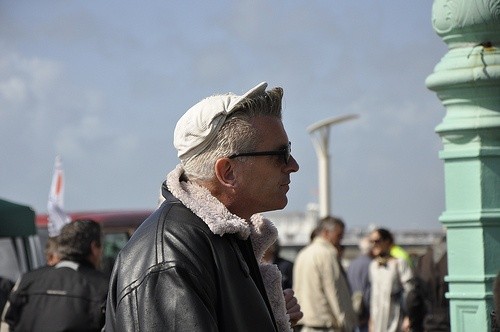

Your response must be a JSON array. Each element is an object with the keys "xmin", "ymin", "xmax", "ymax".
[{"xmin": 228, "ymin": 139, "xmax": 293, "ymax": 164}]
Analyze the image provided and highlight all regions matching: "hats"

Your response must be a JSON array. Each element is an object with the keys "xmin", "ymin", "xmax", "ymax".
[{"xmin": 172, "ymin": 81, "xmax": 270, "ymax": 163}]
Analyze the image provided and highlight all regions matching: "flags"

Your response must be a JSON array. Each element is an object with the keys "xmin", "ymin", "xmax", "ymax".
[{"xmin": 46, "ymin": 154, "xmax": 72, "ymax": 238}]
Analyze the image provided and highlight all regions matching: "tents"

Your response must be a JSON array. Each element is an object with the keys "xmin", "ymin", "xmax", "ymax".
[{"xmin": 0, "ymin": 199, "xmax": 45, "ymax": 283}]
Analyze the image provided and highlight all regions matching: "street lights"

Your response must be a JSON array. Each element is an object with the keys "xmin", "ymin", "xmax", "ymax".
[{"xmin": 309, "ymin": 111, "xmax": 360, "ymax": 221}]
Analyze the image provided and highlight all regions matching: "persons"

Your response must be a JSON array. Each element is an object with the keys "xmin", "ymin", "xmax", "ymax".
[
  {"xmin": 264, "ymin": 214, "xmax": 449, "ymax": 332},
  {"xmin": 101, "ymin": 83, "xmax": 303, "ymax": 332},
  {"xmin": 0, "ymin": 217, "xmax": 110, "ymax": 332}
]
